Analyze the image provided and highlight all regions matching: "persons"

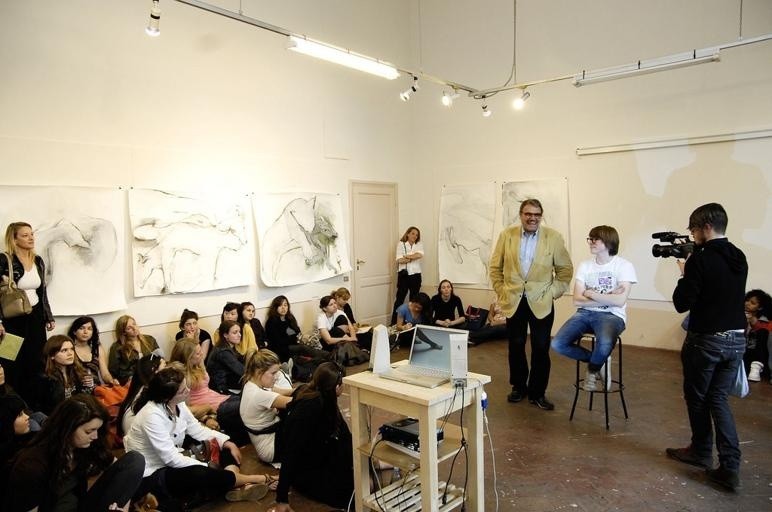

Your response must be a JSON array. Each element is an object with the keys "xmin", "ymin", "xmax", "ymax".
[
  {"xmin": 549, "ymin": 223, "xmax": 638, "ymax": 396},
  {"xmin": 490, "ymin": 200, "xmax": 572, "ymax": 414},
  {"xmin": 0, "ymin": 221, "xmax": 58, "ymax": 412},
  {"xmin": 742, "ymin": 289, "xmax": 772, "ymax": 386},
  {"xmin": 390, "ymin": 226, "xmax": 423, "ymax": 326},
  {"xmin": 661, "ymin": 200, "xmax": 751, "ymax": 495},
  {"xmin": 1, "ymin": 279, "xmax": 465, "ymax": 511}
]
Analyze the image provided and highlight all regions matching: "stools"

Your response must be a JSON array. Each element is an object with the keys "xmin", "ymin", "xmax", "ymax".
[{"xmin": 570, "ymin": 333, "xmax": 628, "ymax": 430}]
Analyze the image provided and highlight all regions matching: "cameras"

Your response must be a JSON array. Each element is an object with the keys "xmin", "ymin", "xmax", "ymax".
[{"xmin": 651, "ymin": 232, "xmax": 696, "ymax": 258}]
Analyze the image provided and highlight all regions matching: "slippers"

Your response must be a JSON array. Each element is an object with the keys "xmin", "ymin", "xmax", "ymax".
[{"xmin": 225, "ymin": 473, "xmax": 279, "ymax": 502}]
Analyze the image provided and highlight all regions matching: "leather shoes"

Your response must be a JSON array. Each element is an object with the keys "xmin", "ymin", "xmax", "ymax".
[
  {"xmin": 508, "ymin": 388, "xmax": 527, "ymax": 403},
  {"xmin": 530, "ymin": 396, "xmax": 553, "ymax": 410}
]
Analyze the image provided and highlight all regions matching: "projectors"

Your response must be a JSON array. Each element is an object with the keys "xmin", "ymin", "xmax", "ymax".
[{"xmin": 381, "ymin": 418, "xmax": 444, "ymax": 451}]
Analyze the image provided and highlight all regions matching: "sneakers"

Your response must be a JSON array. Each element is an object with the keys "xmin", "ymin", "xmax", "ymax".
[
  {"xmin": 704, "ymin": 466, "xmax": 740, "ymax": 490},
  {"xmin": 415, "ymin": 339, "xmax": 422, "ymax": 344},
  {"xmin": 431, "ymin": 344, "xmax": 443, "ymax": 350},
  {"xmin": 583, "ymin": 372, "xmax": 597, "ymax": 392},
  {"xmin": 747, "ymin": 361, "xmax": 764, "ymax": 382},
  {"xmin": 667, "ymin": 446, "xmax": 711, "ymax": 468},
  {"xmin": 600, "ymin": 355, "xmax": 611, "ymax": 391}
]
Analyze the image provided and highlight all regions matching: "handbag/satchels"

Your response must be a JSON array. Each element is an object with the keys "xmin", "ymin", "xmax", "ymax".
[
  {"xmin": 329, "ymin": 341, "xmax": 369, "ymax": 367},
  {"xmin": 466, "ymin": 305, "xmax": 489, "ymax": 328},
  {"xmin": 729, "ymin": 359, "xmax": 749, "ymax": 398},
  {"xmin": 0, "ymin": 286, "xmax": 33, "ymax": 318},
  {"xmin": 296, "ymin": 334, "xmax": 324, "ymax": 351}
]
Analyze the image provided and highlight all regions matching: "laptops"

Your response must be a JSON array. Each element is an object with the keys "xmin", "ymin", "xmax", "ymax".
[{"xmin": 379, "ymin": 324, "xmax": 470, "ymax": 389}]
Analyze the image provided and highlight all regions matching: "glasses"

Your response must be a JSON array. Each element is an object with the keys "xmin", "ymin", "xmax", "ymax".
[{"xmin": 587, "ymin": 238, "xmax": 599, "ymax": 245}]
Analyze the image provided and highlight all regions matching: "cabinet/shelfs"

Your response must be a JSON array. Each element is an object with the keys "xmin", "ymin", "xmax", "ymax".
[{"xmin": 342, "ymin": 360, "xmax": 491, "ymax": 512}]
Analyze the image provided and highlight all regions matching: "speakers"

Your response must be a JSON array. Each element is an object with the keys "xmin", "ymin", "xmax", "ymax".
[
  {"xmin": 367, "ymin": 324, "xmax": 390, "ymax": 371},
  {"xmin": 449, "ymin": 333, "xmax": 468, "ymax": 388}
]
